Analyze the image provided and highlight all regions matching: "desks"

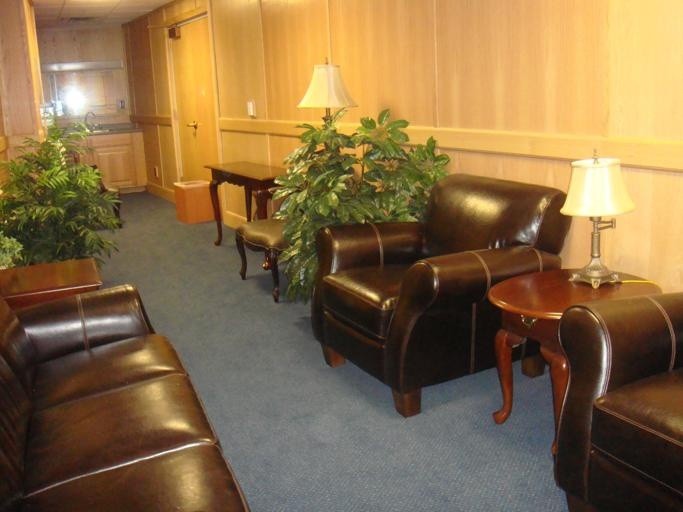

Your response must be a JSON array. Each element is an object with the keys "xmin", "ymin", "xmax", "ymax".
[{"xmin": 202, "ymin": 160, "xmax": 291, "ymax": 250}]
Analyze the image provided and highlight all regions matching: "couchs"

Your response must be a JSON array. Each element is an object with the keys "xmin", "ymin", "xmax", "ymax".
[{"xmin": 0, "ymin": 278, "xmax": 261, "ymax": 512}]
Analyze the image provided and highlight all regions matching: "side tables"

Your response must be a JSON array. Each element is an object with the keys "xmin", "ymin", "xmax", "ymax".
[
  {"xmin": 486, "ymin": 269, "xmax": 664, "ymax": 457},
  {"xmin": 0, "ymin": 258, "xmax": 103, "ymax": 312}
]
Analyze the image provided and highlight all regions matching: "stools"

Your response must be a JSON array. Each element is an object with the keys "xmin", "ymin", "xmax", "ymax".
[{"xmin": 233, "ymin": 221, "xmax": 293, "ymax": 306}]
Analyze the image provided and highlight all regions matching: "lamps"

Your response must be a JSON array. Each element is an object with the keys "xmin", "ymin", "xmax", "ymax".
[
  {"xmin": 558, "ymin": 148, "xmax": 632, "ymax": 293},
  {"xmin": 295, "ymin": 62, "xmax": 358, "ymax": 137}
]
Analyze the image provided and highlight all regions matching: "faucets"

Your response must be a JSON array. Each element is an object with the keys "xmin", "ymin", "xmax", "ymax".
[{"xmin": 84, "ymin": 111, "xmax": 96, "ymax": 126}]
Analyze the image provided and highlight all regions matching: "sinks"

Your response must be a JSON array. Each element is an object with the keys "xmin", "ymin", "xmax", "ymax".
[{"xmin": 68, "ymin": 128, "xmax": 110, "ymax": 135}]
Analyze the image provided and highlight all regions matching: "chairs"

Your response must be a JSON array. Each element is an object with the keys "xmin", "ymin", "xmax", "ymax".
[
  {"xmin": 553, "ymin": 292, "xmax": 683, "ymax": 511},
  {"xmin": 308, "ymin": 173, "xmax": 570, "ymax": 421}
]
